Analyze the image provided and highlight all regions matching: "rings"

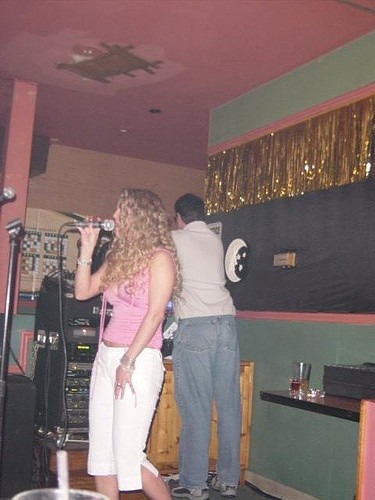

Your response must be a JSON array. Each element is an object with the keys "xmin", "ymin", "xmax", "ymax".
[{"xmin": 116, "ymin": 384, "xmax": 122, "ymax": 389}]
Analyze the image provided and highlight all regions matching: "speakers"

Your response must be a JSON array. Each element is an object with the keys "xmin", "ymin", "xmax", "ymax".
[{"xmin": 0, "ymin": 374, "xmax": 37, "ymax": 500}]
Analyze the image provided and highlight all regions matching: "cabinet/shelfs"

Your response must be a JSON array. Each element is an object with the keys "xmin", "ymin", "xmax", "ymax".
[{"xmin": 146, "ymin": 361, "xmax": 255, "ymax": 485}]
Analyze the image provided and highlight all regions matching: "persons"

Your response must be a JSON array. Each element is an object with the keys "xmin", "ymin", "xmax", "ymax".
[
  {"xmin": 167, "ymin": 193, "xmax": 243, "ymax": 500},
  {"xmin": 71, "ymin": 186, "xmax": 182, "ymax": 500}
]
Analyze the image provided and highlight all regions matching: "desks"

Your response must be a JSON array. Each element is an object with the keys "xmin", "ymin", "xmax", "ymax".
[{"xmin": 258, "ymin": 386, "xmax": 360, "ymax": 423}]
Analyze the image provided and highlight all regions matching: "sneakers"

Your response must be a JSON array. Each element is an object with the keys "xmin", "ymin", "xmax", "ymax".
[
  {"xmin": 208, "ymin": 469, "xmax": 237, "ymax": 497},
  {"xmin": 158, "ymin": 472, "xmax": 207, "ymax": 499}
]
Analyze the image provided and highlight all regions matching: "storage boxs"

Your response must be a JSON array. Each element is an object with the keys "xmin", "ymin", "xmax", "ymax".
[{"xmin": 323, "ymin": 363, "xmax": 375, "ymax": 401}]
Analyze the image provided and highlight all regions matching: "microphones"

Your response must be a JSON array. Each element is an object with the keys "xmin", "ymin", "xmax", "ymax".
[
  {"xmin": 0, "ymin": 186, "xmax": 16, "ymax": 204},
  {"xmin": 66, "ymin": 219, "xmax": 116, "ymax": 232}
]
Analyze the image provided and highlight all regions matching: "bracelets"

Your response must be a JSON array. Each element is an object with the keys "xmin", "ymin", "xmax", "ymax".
[
  {"xmin": 77, "ymin": 258, "xmax": 92, "ymax": 266},
  {"xmin": 119, "ymin": 354, "xmax": 136, "ymax": 371}
]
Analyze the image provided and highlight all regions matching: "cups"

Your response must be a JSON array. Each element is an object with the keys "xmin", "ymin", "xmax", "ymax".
[
  {"xmin": 296, "ymin": 362, "xmax": 311, "ymax": 392},
  {"xmin": 287, "ymin": 376, "xmax": 302, "ymax": 396}
]
